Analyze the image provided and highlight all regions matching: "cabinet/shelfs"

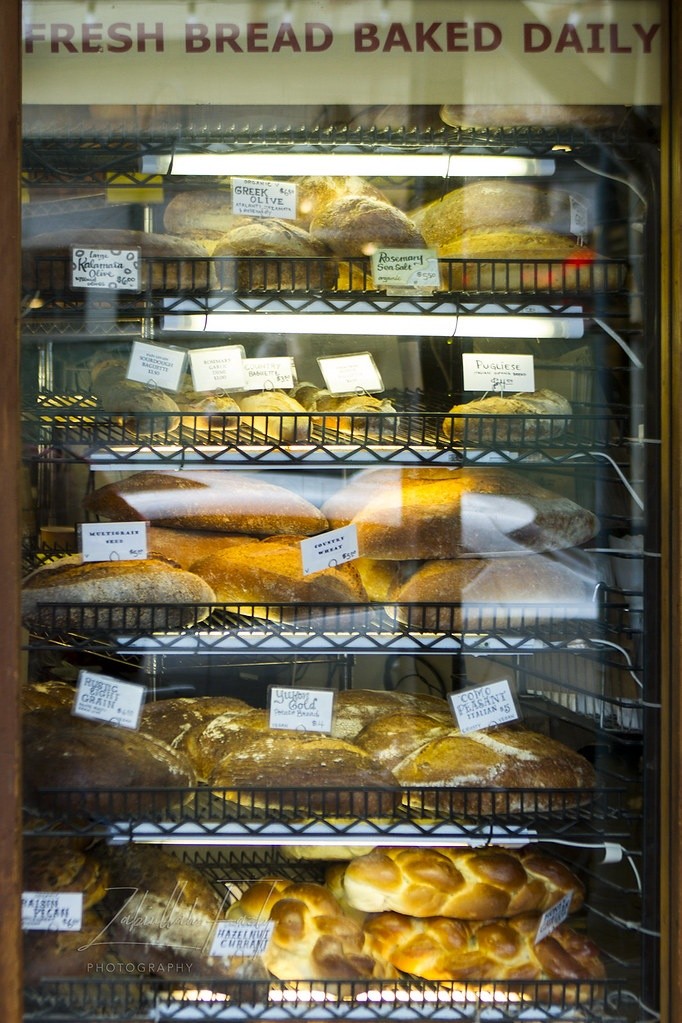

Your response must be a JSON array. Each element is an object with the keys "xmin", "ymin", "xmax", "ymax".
[{"xmin": 11, "ymin": 101, "xmax": 652, "ymax": 1023}]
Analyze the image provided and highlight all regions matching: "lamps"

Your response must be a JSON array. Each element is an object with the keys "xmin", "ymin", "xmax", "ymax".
[{"xmin": 137, "ymin": 150, "xmax": 556, "ymax": 176}]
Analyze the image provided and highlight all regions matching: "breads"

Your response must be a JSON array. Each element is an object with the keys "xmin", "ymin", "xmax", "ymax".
[{"xmin": 17, "ymin": 105, "xmax": 629, "ymax": 1004}]
